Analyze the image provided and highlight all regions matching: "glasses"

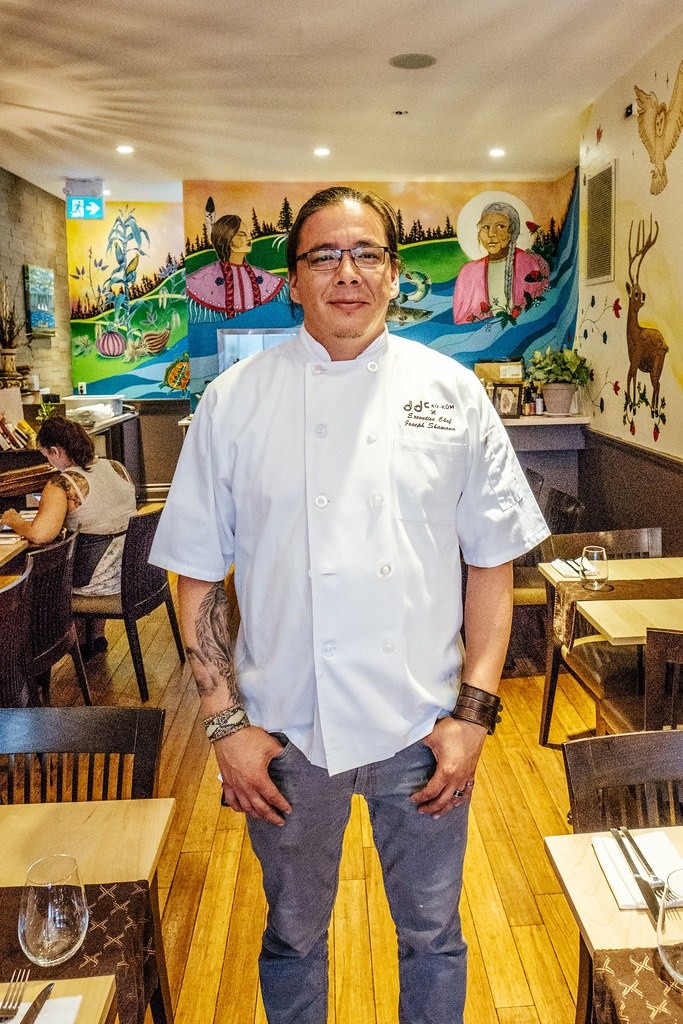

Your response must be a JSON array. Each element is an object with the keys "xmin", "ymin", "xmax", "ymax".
[{"xmin": 295, "ymin": 246, "xmax": 389, "ymax": 272}]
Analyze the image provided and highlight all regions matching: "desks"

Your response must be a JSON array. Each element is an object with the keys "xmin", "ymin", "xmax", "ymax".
[
  {"xmin": 0, "ymin": 798, "xmax": 177, "ymax": 1024},
  {"xmin": 179, "ymin": 414, "xmax": 589, "ymax": 567},
  {"xmin": 0, "ymin": 530, "xmax": 29, "ymax": 567},
  {"xmin": 538, "ymin": 557, "xmax": 683, "ymax": 697},
  {"xmin": 543, "ymin": 825, "xmax": 683, "ymax": 1024}
]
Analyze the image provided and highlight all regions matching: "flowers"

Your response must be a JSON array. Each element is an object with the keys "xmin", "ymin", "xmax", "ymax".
[{"xmin": 527, "ymin": 345, "xmax": 591, "ymax": 394}]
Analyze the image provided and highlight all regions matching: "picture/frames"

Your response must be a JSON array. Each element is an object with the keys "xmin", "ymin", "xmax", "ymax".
[{"xmin": 493, "ymin": 383, "xmax": 523, "ymax": 419}]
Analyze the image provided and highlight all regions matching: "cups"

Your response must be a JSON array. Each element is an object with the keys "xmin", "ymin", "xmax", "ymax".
[
  {"xmin": 579, "ymin": 545, "xmax": 609, "ymax": 591},
  {"xmin": 656, "ymin": 868, "xmax": 683, "ymax": 987},
  {"xmin": 18, "ymin": 853, "xmax": 90, "ymax": 968}
]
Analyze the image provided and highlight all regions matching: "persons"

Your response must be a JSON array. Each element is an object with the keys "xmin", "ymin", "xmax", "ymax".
[
  {"xmin": 147, "ymin": 188, "xmax": 550, "ymax": 1024},
  {"xmin": 1, "ymin": 414, "xmax": 140, "ymax": 660}
]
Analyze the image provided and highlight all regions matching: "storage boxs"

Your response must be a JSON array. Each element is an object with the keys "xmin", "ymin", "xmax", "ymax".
[
  {"xmin": 63, "ymin": 395, "xmax": 124, "ymax": 425},
  {"xmin": 474, "ymin": 358, "xmax": 525, "ymax": 382}
]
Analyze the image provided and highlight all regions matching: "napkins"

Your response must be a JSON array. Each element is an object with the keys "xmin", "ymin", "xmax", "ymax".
[
  {"xmin": 550, "ymin": 557, "xmax": 599, "ymax": 578},
  {"xmin": 591, "ymin": 831, "xmax": 683, "ymax": 909},
  {"xmin": 0, "ymin": 995, "xmax": 82, "ymax": 1024}
]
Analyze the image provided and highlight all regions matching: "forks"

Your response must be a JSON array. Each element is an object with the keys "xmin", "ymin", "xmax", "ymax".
[
  {"xmin": 0, "ymin": 968, "xmax": 31, "ymax": 1023},
  {"xmin": 620, "ymin": 826, "xmax": 678, "ymax": 901}
]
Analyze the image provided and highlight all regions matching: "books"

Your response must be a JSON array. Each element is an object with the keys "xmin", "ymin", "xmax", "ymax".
[{"xmin": 0, "ymin": 421, "xmax": 44, "ymax": 546}]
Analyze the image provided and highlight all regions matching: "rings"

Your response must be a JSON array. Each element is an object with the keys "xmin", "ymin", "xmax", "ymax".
[
  {"xmin": 456, "ymin": 789, "xmax": 465, "ymax": 798},
  {"xmin": 466, "ymin": 781, "xmax": 475, "ymax": 786}
]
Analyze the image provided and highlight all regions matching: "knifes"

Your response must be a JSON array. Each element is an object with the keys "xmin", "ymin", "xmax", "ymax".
[
  {"xmin": 610, "ymin": 828, "xmax": 665, "ymax": 926},
  {"xmin": 20, "ymin": 982, "xmax": 55, "ymax": 1024}
]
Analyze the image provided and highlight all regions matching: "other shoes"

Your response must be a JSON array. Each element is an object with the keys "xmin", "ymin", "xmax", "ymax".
[
  {"xmin": 88, "ymin": 635, "xmax": 108, "ymax": 653},
  {"xmin": 79, "ymin": 643, "xmax": 92, "ymax": 662}
]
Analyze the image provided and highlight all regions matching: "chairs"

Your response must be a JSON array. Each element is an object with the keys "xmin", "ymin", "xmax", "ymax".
[
  {"xmin": 521, "ymin": 467, "xmax": 683, "ymax": 1024},
  {"xmin": 24, "ymin": 523, "xmax": 93, "ymax": 707},
  {"xmin": 0, "ymin": 707, "xmax": 166, "ymax": 800},
  {"xmin": 40, "ymin": 505, "xmax": 184, "ymax": 701},
  {"xmin": 0, "ymin": 556, "xmax": 56, "ymax": 787}
]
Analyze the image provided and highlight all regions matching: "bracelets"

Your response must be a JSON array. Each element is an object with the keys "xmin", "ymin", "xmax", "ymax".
[
  {"xmin": 205, "ymin": 704, "xmax": 251, "ymax": 744},
  {"xmin": 453, "ymin": 684, "xmax": 503, "ymax": 735}
]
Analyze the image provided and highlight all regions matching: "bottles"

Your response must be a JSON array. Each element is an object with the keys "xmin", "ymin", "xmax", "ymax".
[{"xmin": 522, "ymin": 394, "xmax": 544, "ymax": 416}]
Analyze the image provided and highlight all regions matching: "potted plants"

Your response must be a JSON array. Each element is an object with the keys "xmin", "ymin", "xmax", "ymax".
[{"xmin": 36, "ymin": 400, "xmax": 56, "ymax": 432}]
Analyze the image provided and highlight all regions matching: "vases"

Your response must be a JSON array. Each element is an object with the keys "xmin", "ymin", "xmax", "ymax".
[
  {"xmin": 0, "ymin": 348, "xmax": 23, "ymax": 390},
  {"xmin": 540, "ymin": 382, "xmax": 577, "ymax": 414}
]
